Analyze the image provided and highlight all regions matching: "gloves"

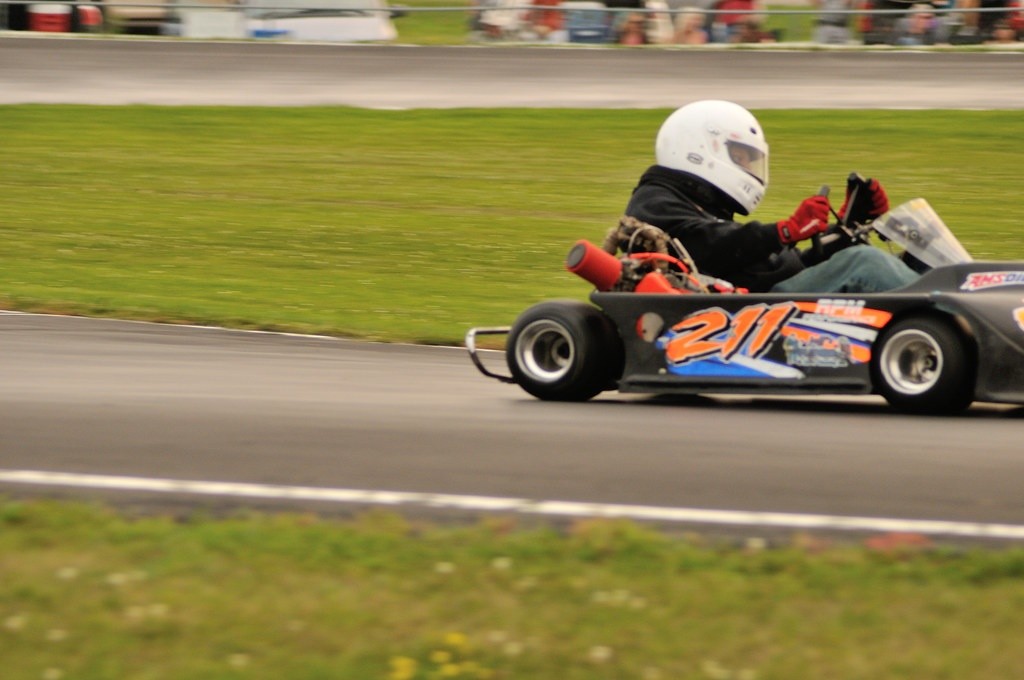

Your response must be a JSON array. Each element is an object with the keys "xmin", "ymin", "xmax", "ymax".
[
  {"xmin": 837, "ymin": 177, "xmax": 889, "ymax": 220},
  {"xmin": 777, "ymin": 196, "xmax": 829, "ymax": 242}
]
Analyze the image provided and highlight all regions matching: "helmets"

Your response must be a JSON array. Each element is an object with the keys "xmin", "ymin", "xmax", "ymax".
[{"xmin": 639, "ymin": 99, "xmax": 768, "ymax": 221}]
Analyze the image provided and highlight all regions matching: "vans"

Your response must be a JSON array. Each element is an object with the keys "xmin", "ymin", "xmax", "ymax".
[
  {"xmin": 157, "ymin": 0, "xmax": 407, "ymax": 44},
  {"xmin": 859, "ymin": 2, "xmax": 987, "ymax": 44}
]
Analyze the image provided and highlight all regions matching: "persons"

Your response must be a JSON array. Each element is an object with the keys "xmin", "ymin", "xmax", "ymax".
[
  {"xmin": 616, "ymin": 99, "xmax": 922, "ymax": 293},
  {"xmin": 465, "ymin": 0, "xmax": 773, "ymax": 46},
  {"xmin": 810, "ymin": 0, "xmax": 1024, "ymax": 46}
]
